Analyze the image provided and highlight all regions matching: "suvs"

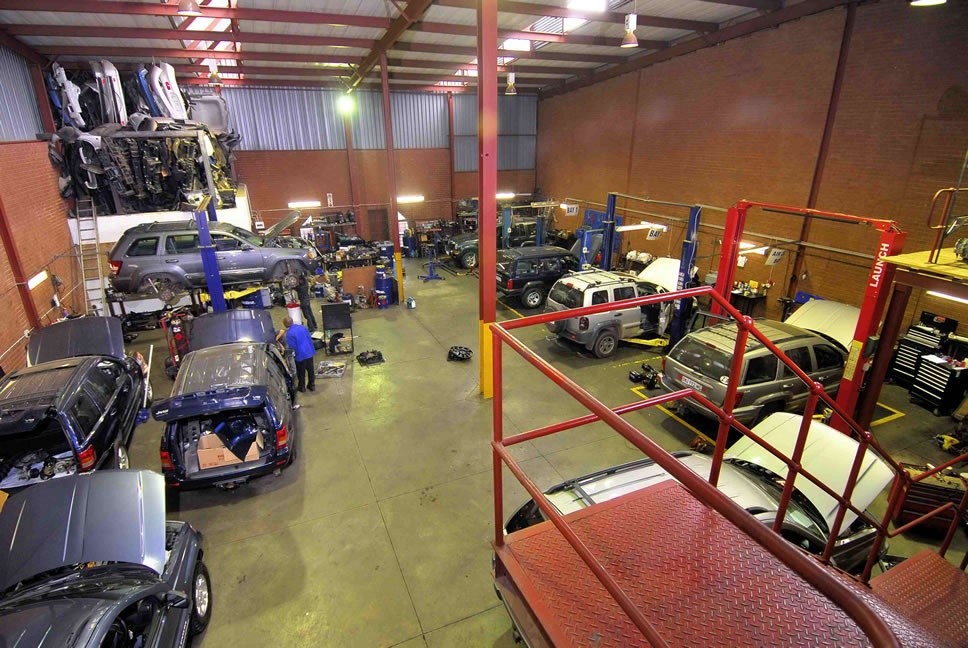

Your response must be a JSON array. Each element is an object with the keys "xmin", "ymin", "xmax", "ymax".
[
  {"xmin": 542, "ymin": 256, "xmax": 699, "ymax": 359},
  {"xmin": 654, "ymin": 315, "xmax": 850, "ymax": 432},
  {"xmin": 499, "ymin": 407, "xmax": 895, "ymax": 581},
  {"xmin": 494, "ymin": 246, "xmax": 582, "ymax": 309},
  {"xmin": 0, "ymin": 470, "xmax": 213, "ymax": 648},
  {"xmin": 153, "ymin": 308, "xmax": 300, "ymax": 496},
  {"xmin": 446, "ymin": 216, "xmax": 548, "ymax": 271},
  {"xmin": 105, "ymin": 219, "xmax": 318, "ymax": 296},
  {"xmin": 0, "ymin": 316, "xmax": 154, "ymax": 503}
]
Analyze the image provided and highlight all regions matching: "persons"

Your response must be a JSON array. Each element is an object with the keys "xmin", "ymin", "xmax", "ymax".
[
  {"xmin": 283, "ymin": 317, "xmax": 316, "ymax": 392},
  {"xmin": 292, "ymin": 275, "xmax": 317, "ymax": 331}
]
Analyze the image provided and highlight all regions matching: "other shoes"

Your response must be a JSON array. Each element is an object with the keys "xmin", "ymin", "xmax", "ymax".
[
  {"xmin": 307, "ymin": 384, "xmax": 315, "ymax": 391},
  {"xmin": 297, "ymin": 387, "xmax": 306, "ymax": 392}
]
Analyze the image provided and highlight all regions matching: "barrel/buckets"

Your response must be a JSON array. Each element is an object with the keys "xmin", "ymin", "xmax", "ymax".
[
  {"xmin": 288, "ymin": 304, "xmax": 303, "ymax": 325},
  {"xmin": 376, "ymin": 276, "xmax": 394, "ymax": 305}
]
